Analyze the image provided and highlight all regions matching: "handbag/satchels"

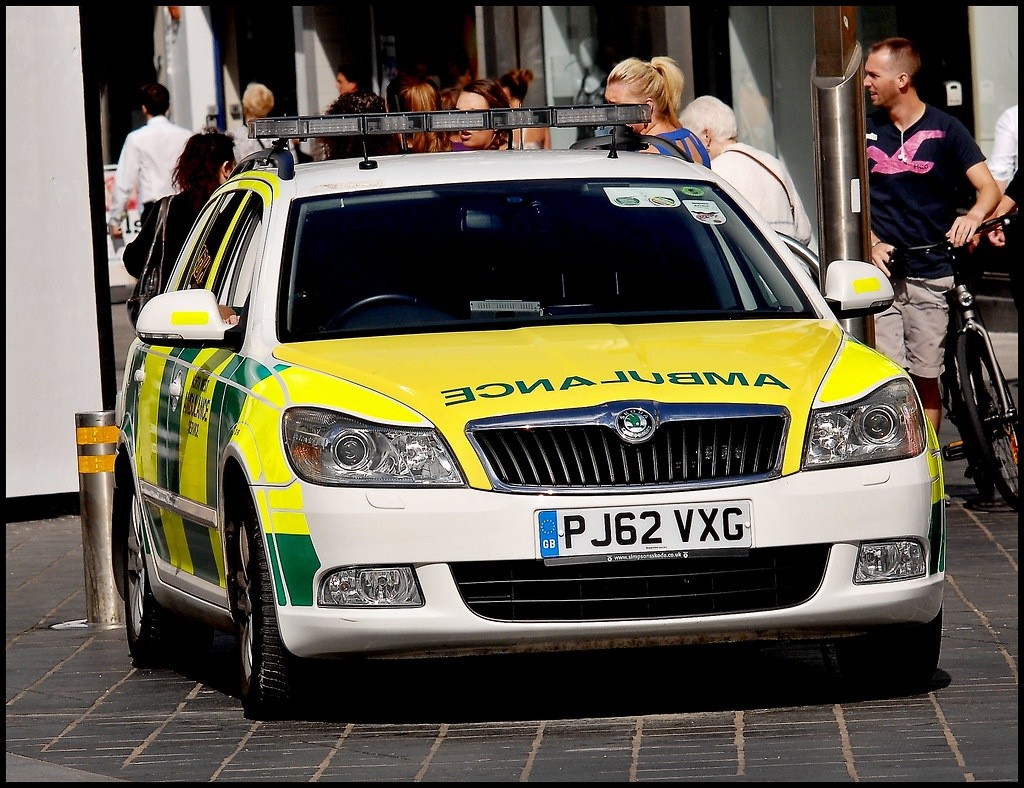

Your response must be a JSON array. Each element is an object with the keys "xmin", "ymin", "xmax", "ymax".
[{"xmin": 125, "ymin": 265, "xmax": 162, "ymax": 330}]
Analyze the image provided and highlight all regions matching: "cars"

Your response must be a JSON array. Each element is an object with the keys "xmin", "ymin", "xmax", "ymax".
[{"xmin": 109, "ymin": 100, "xmax": 948, "ymax": 722}]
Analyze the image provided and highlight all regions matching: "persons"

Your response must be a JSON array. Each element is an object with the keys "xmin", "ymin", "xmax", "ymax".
[
  {"xmin": 107, "ymin": 83, "xmax": 194, "ymax": 234},
  {"xmin": 121, "ymin": 132, "xmax": 240, "ymax": 314},
  {"xmin": 853, "ymin": 33, "xmax": 1003, "ymax": 435},
  {"xmin": 605, "ymin": 52, "xmax": 708, "ymax": 175},
  {"xmin": 985, "ymin": 102, "xmax": 1019, "ymax": 246},
  {"xmin": 678, "ymin": 94, "xmax": 819, "ymax": 262},
  {"xmin": 172, "ymin": 63, "xmax": 548, "ymax": 162}
]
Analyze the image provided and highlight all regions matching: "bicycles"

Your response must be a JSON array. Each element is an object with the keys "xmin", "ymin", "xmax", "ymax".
[{"xmin": 886, "ymin": 207, "xmax": 1017, "ymax": 515}]
